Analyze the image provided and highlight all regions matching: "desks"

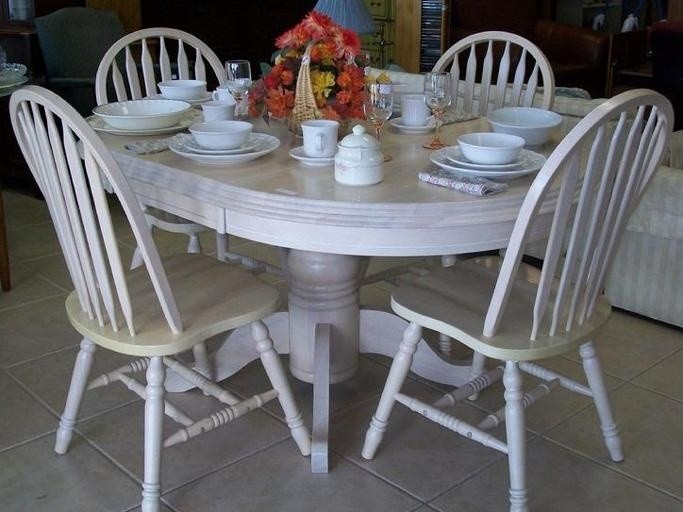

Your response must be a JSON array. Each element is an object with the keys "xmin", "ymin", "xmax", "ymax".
[{"xmin": 75, "ymin": 81, "xmax": 632, "ymax": 478}]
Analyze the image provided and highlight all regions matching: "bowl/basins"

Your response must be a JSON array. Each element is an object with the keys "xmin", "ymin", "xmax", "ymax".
[
  {"xmin": 91, "ymin": 100, "xmax": 191, "ymax": 130},
  {"xmin": 485, "ymin": 107, "xmax": 562, "ymax": 150},
  {"xmin": 392, "ymin": 84, "xmax": 426, "ymax": 106},
  {"xmin": 0, "ymin": 64, "xmax": 26, "ymax": 83},
  {"xmin": 187, "ymin": 121, "xmax": 253, "ymax": 150},
  {"xmin": 156, "ymin": 79, "xmax": 208, "ymax": 101},
  {"xmin": 455, "ymin": 132, "xmax": 526, "ymax": 166}
]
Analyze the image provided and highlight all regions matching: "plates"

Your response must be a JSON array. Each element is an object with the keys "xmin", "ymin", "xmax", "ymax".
[
  {"xmin": 82, "ymin": 114, "xmax": 194, "ymax": 136},
  {"xmin": 287, "ymin": 146, "xmax": 336, "ymax": 168},
  {"xmin": 388, "ymin": 115, "xmax": 441, "ymax": 135},
  {"xmin": 429, "ymin": 144, "xmax": 547, "ymax": 180},
  {"xmin": 166, "ymin": 132, "xmax": 281, "ymax": 167},
  {"xmin": 389, "ymin": 103, "xmax": 401, "ymax": 115},
  {"xmin": 0, "ymin": 76, "xmax": 29, "ymax": 90},
  {"xmin": 143, "ymin": 91, "xmax": 212, "ymax": 104}
]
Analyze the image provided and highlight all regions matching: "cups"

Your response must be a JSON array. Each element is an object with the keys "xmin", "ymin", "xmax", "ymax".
[
  {"xmin": 391, "ymin": 83, "xmax": 408, "ymax": 117},
  {"xmin": 400, "ymin": 94, "xmax": 433, "ymax": 128},
  {"xmin": 211, "ymin": 86, "xmax": 237, "ymax": 116},
  {"xmin": 200, "ymin": 100, "xmax": 236, "ymax": 121},
  {"xmin": 301, "ymin": 119, "xmax": 340, "ymax": 159}
]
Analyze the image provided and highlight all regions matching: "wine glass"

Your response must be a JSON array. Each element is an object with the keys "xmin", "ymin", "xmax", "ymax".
[
  {"xmin": 224, "ymin": 59, "xmax": 252, "ymax": 121},
  {"xmin": 361, "ymin": 82, "xmax": 395, "ymax": 163},
  {"xmin": 424, "ymin": 72, "xmax": 454, "ymax": 151}
]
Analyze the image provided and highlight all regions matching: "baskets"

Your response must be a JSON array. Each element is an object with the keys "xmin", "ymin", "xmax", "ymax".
[{"xmin": 288, "ymin": 40, "xmax": 349, "ymax": 138}]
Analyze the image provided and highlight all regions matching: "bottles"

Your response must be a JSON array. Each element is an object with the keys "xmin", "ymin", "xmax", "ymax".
[{"xmin": 333, "ymin": 125, "xmax": 383, "ymax": 186}]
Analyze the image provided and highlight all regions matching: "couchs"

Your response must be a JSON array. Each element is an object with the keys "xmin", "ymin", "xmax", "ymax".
[
  {"xmin": 602, "ymin": 130, "xmax": 682, "ymax": 335},
  {"xmin": 427, "ymin": 68, "xmax": 639, "ymax": 268}
]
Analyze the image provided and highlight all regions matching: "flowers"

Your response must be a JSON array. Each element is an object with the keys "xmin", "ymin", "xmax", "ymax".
[{"xmin": 239, "ymin": 10, "xmax": 375, "ymax": 133}]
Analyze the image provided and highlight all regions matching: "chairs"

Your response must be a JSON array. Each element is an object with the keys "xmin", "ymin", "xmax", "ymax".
[
  {"xmin": 8, "ymin": 81, "xmax": 318, "ymax": 512},
  {"xmin": 357, "ymin": 86, "xmax": 675, "ymax": 511},
  {"xmin": 30, "ymin": 6, "xmax": 196, "ymax": 117},
  {"xmin": 362, "ymin": 27, "xmax": 554, "ymax": 356},
  {"xmin": 93, "ymin": 27, "xmax": 288, "ymax": 301},
  {"xmin": 525, "ymin": 21, "xmax": 621, "ymax": 97}
]
